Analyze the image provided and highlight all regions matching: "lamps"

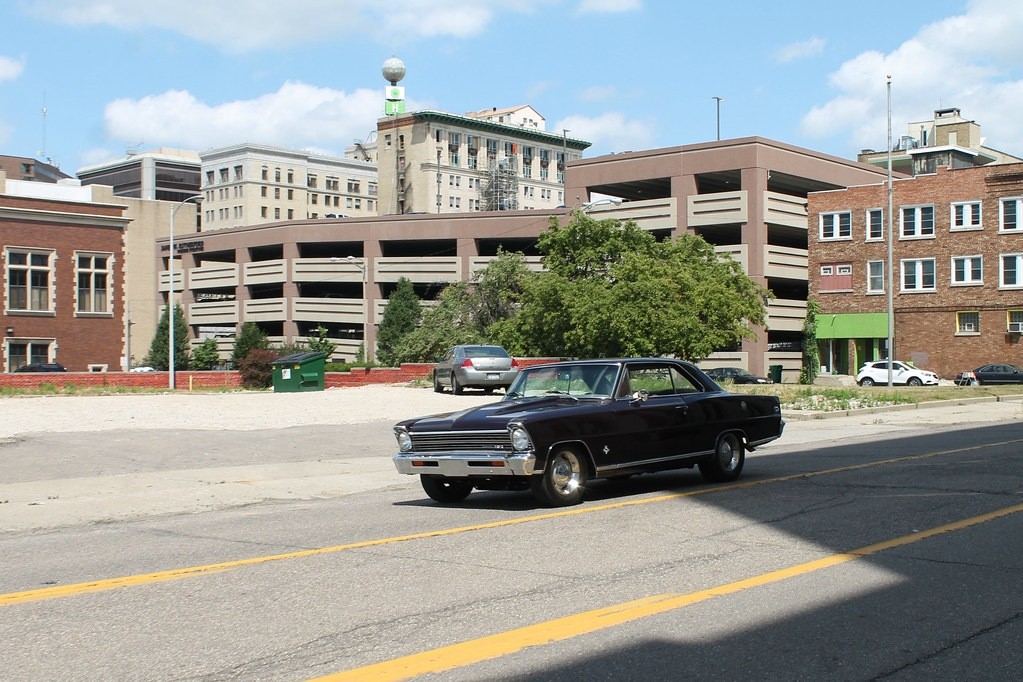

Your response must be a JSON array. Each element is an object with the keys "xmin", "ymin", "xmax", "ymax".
[{"xmin": 7, "ymin": 327, "xmax": 13, "ymax": 333}]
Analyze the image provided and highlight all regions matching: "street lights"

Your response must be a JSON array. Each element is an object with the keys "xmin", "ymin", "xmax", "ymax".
[
  {"xmin": 169, "ymin": 195, "xmax": 206, "ymax": 389},
  {"xmin": 563, "ymin": 128, "xmax": 571, "ymax": 169},
  {"xmin": 886, "ymin": 74, "xmax": 893, "ymax": 386},
  {"xmin": 712, "ymin": 96, "xmax": 725, "ymax": 140}
]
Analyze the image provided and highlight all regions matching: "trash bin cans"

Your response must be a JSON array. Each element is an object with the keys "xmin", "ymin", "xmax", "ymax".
[
  {"xmin": 272, "ymin": 351, "xmax": 328, "ymax": 392},
  {"xmin": 769, "ymin": 365, "xmax": 783, "ymax": 383}
]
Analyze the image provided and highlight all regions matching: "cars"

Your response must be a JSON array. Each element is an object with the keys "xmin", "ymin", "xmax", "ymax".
[
  {"xmin": 14, "ymin": 362, "xmax": 68, "ymax": 373},
  {"xmin": 392, "ymin": 357, "xmax": 787, "ymax": 508},
  {"xmin": 855, "ymin": 360, "xmax": 940, "ymax": 386},
  {"xmin": 704, "ymin": 367, "xmax": 775, "ymax": 384},
  {"xmin": 129, "ymin": 366, "xmax": 154, "ymax": 373},
  {"xmin": 954, "ymin": 363, "xmax": 1022, "ymax": 385},
  {"xmin": 432, "ymin": 345, "xmax": 520, "ymax": 396}
]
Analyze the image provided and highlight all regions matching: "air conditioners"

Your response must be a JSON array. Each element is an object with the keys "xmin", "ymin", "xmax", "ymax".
[{"xmin": 1007, "ymin": 322, "xmax": 1023, "ymax": 332}]
